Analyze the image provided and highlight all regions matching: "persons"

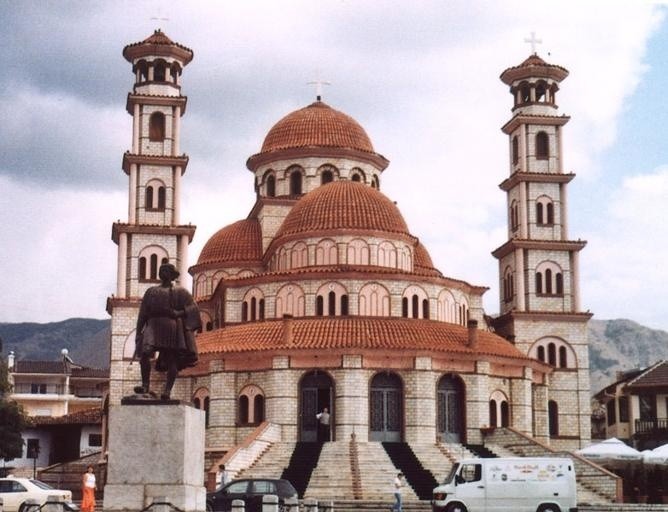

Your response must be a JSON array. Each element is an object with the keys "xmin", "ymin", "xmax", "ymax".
[
  {"xmin": 219, "ymin": 464, "xmax": 227, "ymax": 488},
  {"xmin": 390, "ymin": 473, "xmax": 405, "ymax": 512},
  {"xmin": 132, "ymin": 263, "xmax": 203, "ymax": 399},
  {"xmin": 318, "ymin": 408, "xmax": 330, "ymax": 440},
  {"xmin": 80, "ymin": 464, "xmax": 98, "ymax": 512}
]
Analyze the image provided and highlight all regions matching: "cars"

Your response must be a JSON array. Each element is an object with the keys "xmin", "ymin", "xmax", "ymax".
[
  {"xmin": 0, "ymin": 477, "xmax": 73, "ymax": 512},
  {"xmin": 206, "ymin": 478, "xmax": 298, "ymax": 512}
]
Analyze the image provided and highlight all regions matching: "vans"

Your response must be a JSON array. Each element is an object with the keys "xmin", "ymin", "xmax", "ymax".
[{"xmin": 429, "ymin": 457, "xmax": 578, "ymax": 512}]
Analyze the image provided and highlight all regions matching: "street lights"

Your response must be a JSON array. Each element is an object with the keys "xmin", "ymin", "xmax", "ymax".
[{"xmin": 61, "ymin": 348, "xmax": 74, "ymax": 415}]
